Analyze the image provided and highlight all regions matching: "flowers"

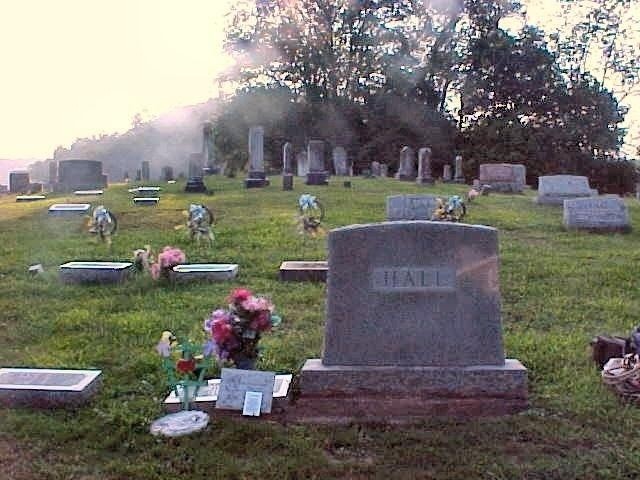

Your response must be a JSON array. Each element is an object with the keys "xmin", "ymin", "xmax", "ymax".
[
  {"xmin": 151, "ymin": 246, "xmax": 185, "ymax": 283},
  {"xmin": 200, "ymin": 288, "xmax": 282, "ymax": 365}
]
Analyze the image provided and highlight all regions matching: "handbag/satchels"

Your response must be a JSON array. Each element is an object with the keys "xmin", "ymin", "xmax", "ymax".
[{"xmin": 592, "ymin": 326, "xmax": 638, "ymax": 399}]
